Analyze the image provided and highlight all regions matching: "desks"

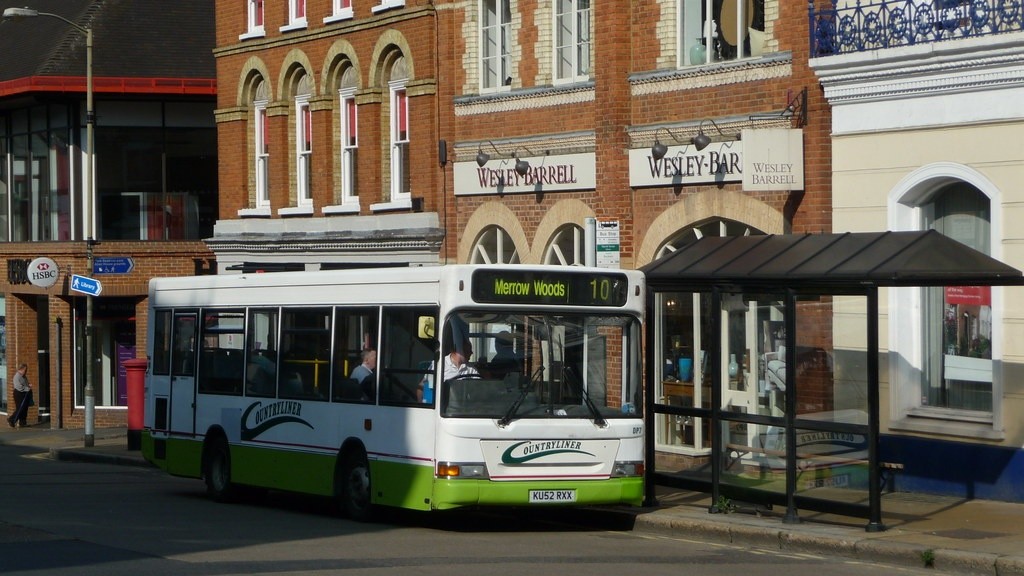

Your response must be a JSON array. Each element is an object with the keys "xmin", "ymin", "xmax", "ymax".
[{"xmin": 663, "ymin": 382, "xmax": 712, "ymax": 448}]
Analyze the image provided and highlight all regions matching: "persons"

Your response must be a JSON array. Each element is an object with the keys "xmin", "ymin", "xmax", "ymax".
[
  {"xmin": 417, "ymin": 340, "xmax": 481, "ymax": 404},
  {"xmin": 490, "ymin": 331, "xmax": 523, "ymax": 379},
  {"xmin": 350, "ymin": 348, "xmax": 377, "ymax": 385},
  {"xmin": 7, "ymin": 363, "xmax": 34, "ymax": 427}
]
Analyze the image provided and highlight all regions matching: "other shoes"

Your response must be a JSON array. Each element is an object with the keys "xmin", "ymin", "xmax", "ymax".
[
  {"xmin": 7, "ymin": 418, "xmax": 15, "ymax": 428},
  {"xmin": 18, "ymin": 424, "xmax": 31, "ymax": 428}
]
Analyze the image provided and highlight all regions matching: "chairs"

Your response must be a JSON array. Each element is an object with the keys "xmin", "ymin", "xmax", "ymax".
[{"xmin": 174, "ymin": 349, "xmax": 431, "ymax": 403}]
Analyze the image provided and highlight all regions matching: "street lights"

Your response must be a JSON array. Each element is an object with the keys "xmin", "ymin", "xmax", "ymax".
[{"xmin": 1, "ymin": 7, "xmax": 98, "ymax": 446}]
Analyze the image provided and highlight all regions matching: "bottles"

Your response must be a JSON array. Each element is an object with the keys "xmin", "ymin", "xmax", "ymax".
[{"xmin": 728, "ymin": 353, "xmax": 739, "ymax": 377}]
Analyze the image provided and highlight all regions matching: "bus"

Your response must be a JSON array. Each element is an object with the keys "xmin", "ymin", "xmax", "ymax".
[{"xmin": 140, "ymin": 266, "xmax": 648, "ymax": 525}]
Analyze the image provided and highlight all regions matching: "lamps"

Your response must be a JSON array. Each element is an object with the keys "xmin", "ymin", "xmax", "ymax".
[
  {"xmin": 652, "ymin": 126, "xmax": 694, "ymax": 160},
  {"xmin": 513, "ymin": 145, "xmax": 550, "ymax": 176},
  {"xmin": 694, "ymin": 118, "xmax": 741, "ymax": 151},
  {"xmin": 476, "ymin": 139, "xmax": 515, "ymax": 168}
]
